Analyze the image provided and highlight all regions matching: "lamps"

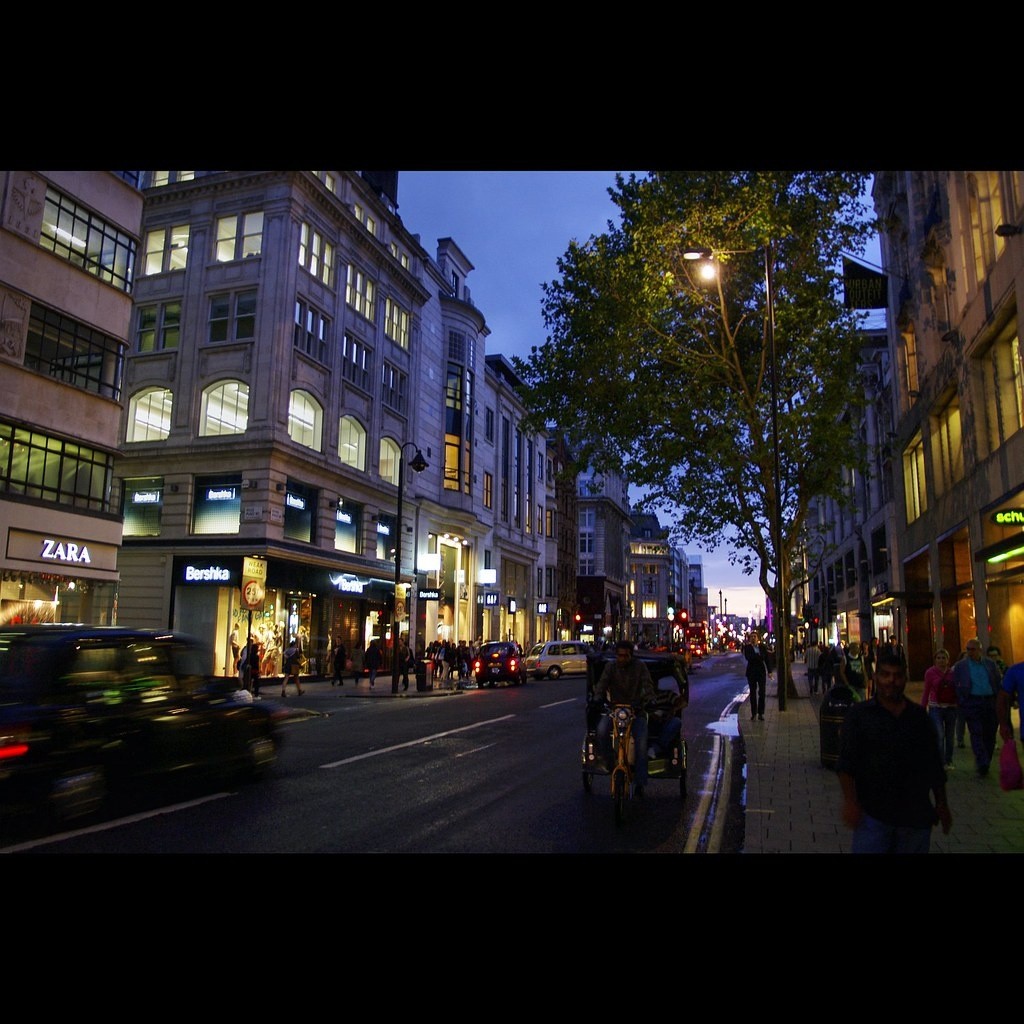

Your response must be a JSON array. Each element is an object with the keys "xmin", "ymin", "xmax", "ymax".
[
  {"xmin": 170, "ymin": 485, "xmax": 179, "ymax": 491},
  {"xmin": 372, "ymin": 515, "xmax": 379, "ymax": 522},
  {"xmin": 276, "ymin": 484, "xmax": 285, "ymax": 491},
  {"xmin": 68, "ymin": 579, "xmax": 77, "ymax": 589},
  {"xmin": 330, "ymin": 497, "xmax": 343, "ymax": 510},
  {"xmin": 407, "ymin": 526, "xmax": 414, "ymax": 532}
]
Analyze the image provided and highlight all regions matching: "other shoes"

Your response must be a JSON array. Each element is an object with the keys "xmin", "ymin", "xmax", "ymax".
[
  {"xmin": 298, "ymin": 690, "xmax": 305, "ymax": 696},
  {"xmin": 958, "ymin": 742, "xmax": 966, "ymax": 749},
  {"xmin": 945, "ymin": 762, "xmax": 955, "ymax": 771},
  {"xmin": 750, "ymin": 716, "xmax": 756, "ymax": 722},
  {"xmin": 369, "ymin": 684, "xmax": 375, "ymax": 689},
  {"xmin": 281, "ymin": 691, "xmax": 287, "ymax": 698},
  {"xmin": 330, "ymin": 680, "xmax": 334, "ymax": 687},
  {"xmin": 337, "ymin": 683, "xmax": 343, "ymax": 687},
  {"xmin": 403, "ymin": 686, "xmax": 408, "ymax": 691},
  {"xmin": 758, "ymin": 714, "xmax": 765, "ymax": 721}
]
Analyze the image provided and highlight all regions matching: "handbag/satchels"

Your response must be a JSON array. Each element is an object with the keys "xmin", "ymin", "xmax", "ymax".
[{"xmin": 998, "ymin": 739, "xmax": 1024, "ymax": 790}]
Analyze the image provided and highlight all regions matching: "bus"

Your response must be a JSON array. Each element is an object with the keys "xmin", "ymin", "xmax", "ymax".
[{"xmin": 684, "ymin": 621, "xmax": 710, "ymax": 656}]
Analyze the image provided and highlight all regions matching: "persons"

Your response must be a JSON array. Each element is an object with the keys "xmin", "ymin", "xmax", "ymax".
[
  {"xmin": 804, "ymin": 636, "xmax": 907, "ymax": 701},
  {"xmin": 744, "ymin": 632, "xmax": 772, "ymax": 720},
  {"xmin": 795, "ymin": 642, "xmax": 803, "ymax": 656},
  {"xmin": 719, "ymin": 640, "xmax": 751, "ymax": 655},
  {"xmin": 838, "ymin": 652, "xmax": 952, "ymax": 853},
  {"xmin": 252, "ymin": 624, "xmax": 268, "ymax": 656},
  {"xmin": 261, "ymin": 621, "xmax": 282, "ymax": 677},
  {"xmin": 331, "ymin": 636, "xmax": 383, "ymax": 689},
  {"xmin": 685, "ymin": 649, "xmax": 694, "ymax": 675},
  {"xmin": 510, "ymin": 641, "xmax": 532, "ymax": 658},
  {"xmin": 399, "ymin": 638, "xmax": 415, "ymax": 691},
  {"xmin": 583, "ymin": 640, "xmax": 657, "ymax": 651},
  {"xmin": 240, "ymin": 639, "xmax": 262, "ymax": 700},
  {"xmin": 426, "ymin": 635, "xmax": 483, "ymax": 681},
  {"xmin": 922, "ymin": 638, "xmax": 1024, "ymax": 776},
  {"xmin": 230, "ymin": 623, "xmax": 240, "ymax": 677},
  {"xmin": 592, "ymin": 638, "xmax": 689, "ymax": 801},
  {"xmin": 280, "ymin": 641, "xmax": 305, "ymax": 698}
]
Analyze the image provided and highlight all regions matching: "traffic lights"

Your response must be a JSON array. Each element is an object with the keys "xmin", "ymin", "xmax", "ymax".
[
  {"xmin": 805, "ymin": 607, "xmax": 819, "ymax": 629},
  {"xmin": 824, "ymin": 596, "xmax": 838, "ymax": 623}
]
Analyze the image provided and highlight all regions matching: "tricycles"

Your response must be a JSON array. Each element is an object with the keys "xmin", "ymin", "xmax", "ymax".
[{"xmin": 580, "ymin": 650, "xmax": 688, "ymax": 828}]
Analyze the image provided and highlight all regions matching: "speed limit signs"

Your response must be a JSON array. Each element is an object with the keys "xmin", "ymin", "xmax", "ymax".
[{"xmin": 242, "ymin": 580, "xmax": 263, "ymax": 606}]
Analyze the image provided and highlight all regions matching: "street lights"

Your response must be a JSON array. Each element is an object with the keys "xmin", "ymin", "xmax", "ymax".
[
  {"xmin": 683, "ymin": 245, "xmax": 788, "ymax": 712},
  {"xmin": 392, "ymin": 440, "xmax": 430, "ymax": 695}
]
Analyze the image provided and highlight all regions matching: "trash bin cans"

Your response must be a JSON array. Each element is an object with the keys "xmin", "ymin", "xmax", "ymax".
[
  {"xmin": 417, "ymin": 656, "xmax": 434, "ymax": 692},
  {"xmin": 819, "ymin": 681, "xmax": 860, "ymax": 767}
]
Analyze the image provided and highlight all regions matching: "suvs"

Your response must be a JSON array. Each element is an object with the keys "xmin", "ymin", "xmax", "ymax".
[{"xmin": 0, "ymin": 621, "xmax": 293, "ymax": 833}]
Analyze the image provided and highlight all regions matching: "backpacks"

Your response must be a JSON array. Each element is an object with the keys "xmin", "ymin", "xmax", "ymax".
[
  {"xmin": 406, "ymin": 647, "xmax": 415, "ymax": 668},
  {"xmin": 936, "ymin": 679, "xmax": 955, "ymax": 703}
]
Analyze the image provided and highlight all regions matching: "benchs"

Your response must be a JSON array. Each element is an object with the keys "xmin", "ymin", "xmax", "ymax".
[{"xmin": 586, "ymin": 690, "xmax": 683, "ymax": 713}]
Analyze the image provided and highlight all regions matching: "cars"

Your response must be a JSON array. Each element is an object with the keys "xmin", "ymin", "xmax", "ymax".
[
  {"xmin": 474, "ymin": 641, "xmax": 527, "ymax": 690},
  {"xmin": 525, "ymin": 640, "xmax": 597, "ymax": 681}
]
